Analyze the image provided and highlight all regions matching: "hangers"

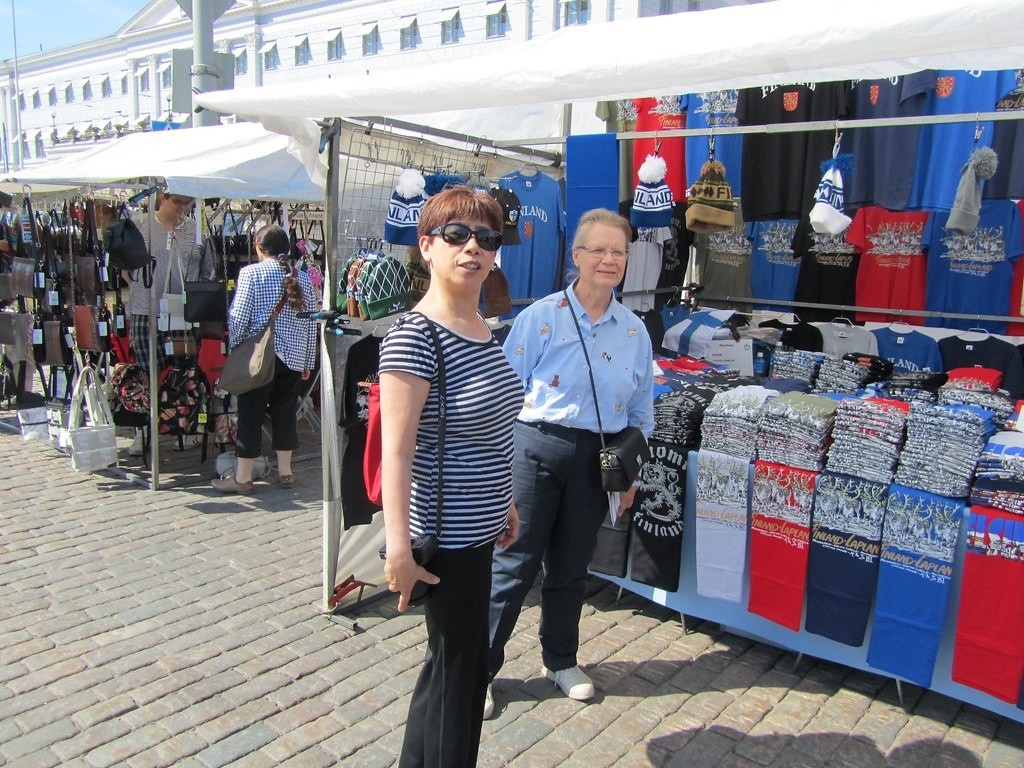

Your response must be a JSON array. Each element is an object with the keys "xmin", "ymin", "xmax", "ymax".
[
  {"xmin": 778, "ymin": 301, "xmax": 801, "ymax": 324},
  {"xmin": 956, "ymin": 315, "xmax": 990, "ymax": 339},
  {"xmin": 722, "ymin": 297, "xmax": 737, "ymax": 311},
  {"xmin": 666, "ymin": 285, "xmax": 681, "ymax": 307},
  {"xmin": 888, "ymin": 308, "xmax": 914, "ymax": 332},
  {"xmin": 832, "ymin": 306, "xmax": 853, "ymax": 326},
  {"xmin": 520, "ymin": 149, "xmax": 539, "ymax": 175},
  {"xmin": 636, "ymin": 289, "xmax": 653, "ymax": 312}
]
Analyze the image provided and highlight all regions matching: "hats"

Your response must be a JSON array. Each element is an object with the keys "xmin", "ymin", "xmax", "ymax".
[
  {"xmin": 945, "ymin": 146, "xmax": 997, "ymax": 235},
  {"xmin": 629, "ymin": 147, "xmax": 737, "ymax": 235},
  {"xmin": 339, "ymin": 169, "xmax": 522, "ymax": 317},
  {"xmin": 809, "ymin": 154, "xmax": 856, "ymax": 236}
]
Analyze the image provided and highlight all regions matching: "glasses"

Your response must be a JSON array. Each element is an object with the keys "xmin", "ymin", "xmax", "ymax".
[
  {"xmin": 578, "ymin": 245, "xmax": 631, "ymax": 261},
  {"xmin": 166, "ymin": 197, "xmax": 192, "ymax": 205},
  {"xmin": 428, "ymin": 223, "xmax": 504, "ymax": 251}
]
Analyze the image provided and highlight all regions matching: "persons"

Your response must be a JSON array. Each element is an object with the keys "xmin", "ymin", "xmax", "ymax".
[
  {"xmin": 482, "ymin": 207, "xmax": 655, "ymax": 720},
  {"xmin": 378, "ymin": 188, "xmax": 525, "ymax": 768},
  {"xmin": 119, "ymin": 193, "xmax": 206, "ymax": 455},
  {"xmin": 211, "ymin": 225, "xmax": 317, "ymax": 494}
]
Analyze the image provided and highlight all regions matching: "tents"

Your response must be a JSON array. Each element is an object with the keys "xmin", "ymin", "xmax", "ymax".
[
  {"xmin": 0, "ymin": 122, "xmax": 329, "ymax": 491},
  {"xmin": 193, "ymin": 0, "xmax": 1024, "ymax": 628}
]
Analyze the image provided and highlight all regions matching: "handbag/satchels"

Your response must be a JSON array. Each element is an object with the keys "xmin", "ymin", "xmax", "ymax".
[
  {"xmin": 207, "ymin": 394, "xmax": 275, "ymax": 479},
  {"xmin": 598, "ymin": 426, "xmax": 652, "ymax": 493},
  {"xmin": 0, "ymin": 191, "xmax": 213, "ymax": 475},
  {"xmin": 198, "ymin": 197, "xmax": 326, "ymax": 305},
  {"xmin": 219, "ymin": 321, "xmax": 277, "ymax": 396},
  {"xmin": 358, "ymin": 319, "xmax": 414, "ymax": 508}
]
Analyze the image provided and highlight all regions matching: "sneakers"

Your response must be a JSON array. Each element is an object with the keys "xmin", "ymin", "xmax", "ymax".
[
  {"xmin": 482, "ymin": 683, "xmax": 495, "ymax": 720},
  {"xmin": 542, "ymin": 663, "xmax": 595, "ymax": 701}
]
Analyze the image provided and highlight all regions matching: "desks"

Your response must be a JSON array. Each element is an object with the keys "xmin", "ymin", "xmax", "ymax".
[{"xmin": 357, "ymin": 381, "xmax": 1024, "ymax": 725}]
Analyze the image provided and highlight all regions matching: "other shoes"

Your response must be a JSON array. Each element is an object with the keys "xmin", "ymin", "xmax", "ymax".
[
  {"xmin": 279, "ymin": 472, "xmax": 297, "ymax": 489},
  {"xmin": 211, "ymin": 473, "xmax": 255, "ymax": 495},
  {"xmin": 128, "ymin": 426, "xmax": 148, "ymax": 456},
  {"xmin": 172, "ymin": 435, "xmax": 204, "ymax": 450}
]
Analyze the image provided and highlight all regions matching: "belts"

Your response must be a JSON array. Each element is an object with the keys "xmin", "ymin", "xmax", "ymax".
[{"xmin": 514, "ymin": 418, "xmax": 613, "ymax": 443}]
